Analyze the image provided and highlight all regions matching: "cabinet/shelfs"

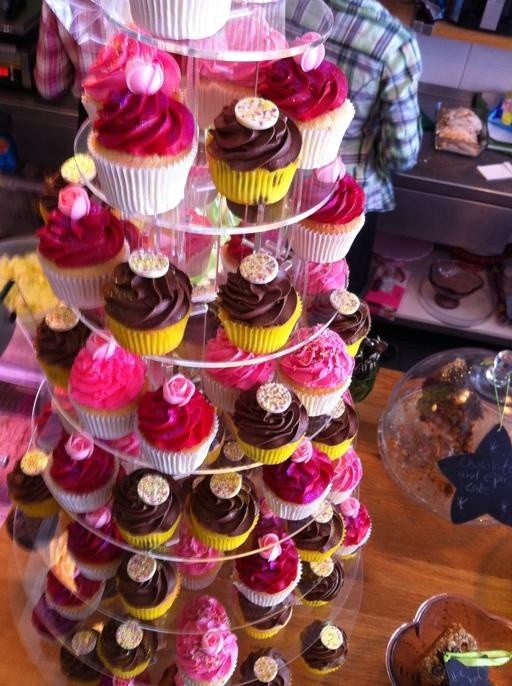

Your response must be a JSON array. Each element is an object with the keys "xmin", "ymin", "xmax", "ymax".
[
  {"xmin": 0, "ymin": 2, "xmax": 373, "ymax": 685},
  {"xmin": 359, "ymin": 80, "xmax": 508, "ymax": 395}
]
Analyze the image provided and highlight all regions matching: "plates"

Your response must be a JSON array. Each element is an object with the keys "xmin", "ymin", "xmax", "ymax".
[{"xmin": 417, "ymin": 274, "xmax": 498, "ymax": 329}]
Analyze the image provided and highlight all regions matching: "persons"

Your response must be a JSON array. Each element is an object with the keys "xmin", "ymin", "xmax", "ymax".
[{"xmin": 266, "ymin": 1, "xmax": 424, "ymax": 291}]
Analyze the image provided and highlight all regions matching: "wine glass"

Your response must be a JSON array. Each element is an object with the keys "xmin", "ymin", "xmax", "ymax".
[{"xmin": 428, "ymin": 260, "xmax": 484, "ymax": 308}]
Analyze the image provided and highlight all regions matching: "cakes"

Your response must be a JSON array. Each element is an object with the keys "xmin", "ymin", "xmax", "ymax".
[
  {"xmin": 419, "ymin": 358, "xmax": 483, "ymax": 454},
  {"xmin": 434, "ymin": 102, "xmax": 484, "ymax": 157}
]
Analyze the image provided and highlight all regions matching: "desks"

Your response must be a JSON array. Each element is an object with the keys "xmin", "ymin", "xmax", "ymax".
[{"xmin": 0, "ymin": 372, "xmax": 512, "ymax": 685}]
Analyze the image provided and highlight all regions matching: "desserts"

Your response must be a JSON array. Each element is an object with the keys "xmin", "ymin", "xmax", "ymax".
[{"xmin": 6, "ymin": 1, "xmax": 375, "ymax": 686}]
